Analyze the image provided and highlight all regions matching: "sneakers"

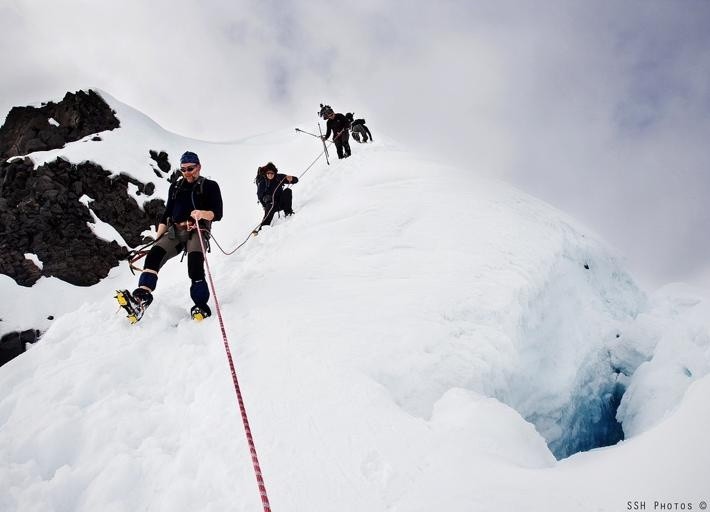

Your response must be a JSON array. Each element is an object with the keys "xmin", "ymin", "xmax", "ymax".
[{"xmin": 286, "ymin": 209, "xmax": 295, "ymax": 216}]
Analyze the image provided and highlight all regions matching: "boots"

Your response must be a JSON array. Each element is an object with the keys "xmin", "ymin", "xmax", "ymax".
[
  {"xmin": 191, "ymin": 303, "xmax": 210, "ymax": 318},
  {"xmin": 124, "ymin": 289, "xmax": 153, "ymax": 320}
]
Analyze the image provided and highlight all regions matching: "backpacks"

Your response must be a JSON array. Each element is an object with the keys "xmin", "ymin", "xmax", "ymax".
[{"xmin": 256, "ymin": 167, "xmax": 271, "ymax": 192}]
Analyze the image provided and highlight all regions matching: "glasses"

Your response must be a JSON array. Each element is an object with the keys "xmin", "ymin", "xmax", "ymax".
[{"xmin": 180, "ymin": 166, "xmax": 195, "ymax": 172}]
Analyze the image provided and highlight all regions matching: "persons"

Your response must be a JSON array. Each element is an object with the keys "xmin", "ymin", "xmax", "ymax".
[
  {"xmin": 252, "ymin": 161, "xmax": 298, "ymax": 236},
  {"xmin": 318, "ymin": 103, "xmax": 373, "ymax": 159},
  {"xmin": 114, "ymin": 151, "xmax": 223, "ymax": 325}
]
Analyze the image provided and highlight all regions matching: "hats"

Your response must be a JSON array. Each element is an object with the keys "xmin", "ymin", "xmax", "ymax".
[
  {"xmin": 264, "ymin": 163, "xmax": 277, "ymax": 173},
  {"xmin": 325, "ymin": 108, "xmax": 333, "ymax": 115},
  {"xmin": 180, "ymin": 151, "xmax": 199, "ymax": 164}
]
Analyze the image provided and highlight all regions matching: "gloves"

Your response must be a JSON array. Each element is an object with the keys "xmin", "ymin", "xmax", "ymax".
[
  {"xmin": 263, "ymin": 195, "xmax": 271, "ymax": 202},
  {"xmin": 140, "ymin": 225, "xmax": 159, "ymax": 240}
]
